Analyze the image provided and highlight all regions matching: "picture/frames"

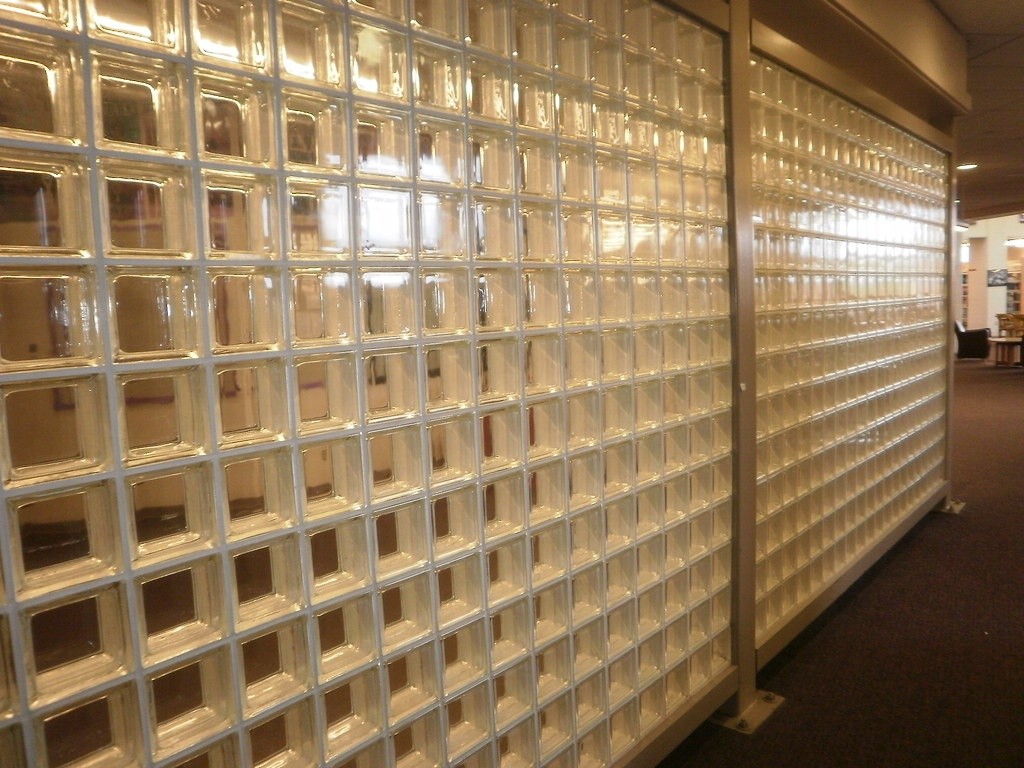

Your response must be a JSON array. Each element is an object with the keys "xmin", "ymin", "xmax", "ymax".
[{"xmin": 40, "ymin": 220, "xmax": 239, "ymax": 412}]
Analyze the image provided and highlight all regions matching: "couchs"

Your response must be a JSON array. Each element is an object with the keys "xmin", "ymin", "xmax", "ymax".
[{"xmin": 955, "ymin": 319, "xmax": 991, "ymax": 359}]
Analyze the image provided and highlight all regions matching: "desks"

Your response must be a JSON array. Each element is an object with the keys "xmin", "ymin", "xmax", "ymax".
[{"xmin": 988, "ymin": 337, "xmax": 1022, "ymax": 367}]
